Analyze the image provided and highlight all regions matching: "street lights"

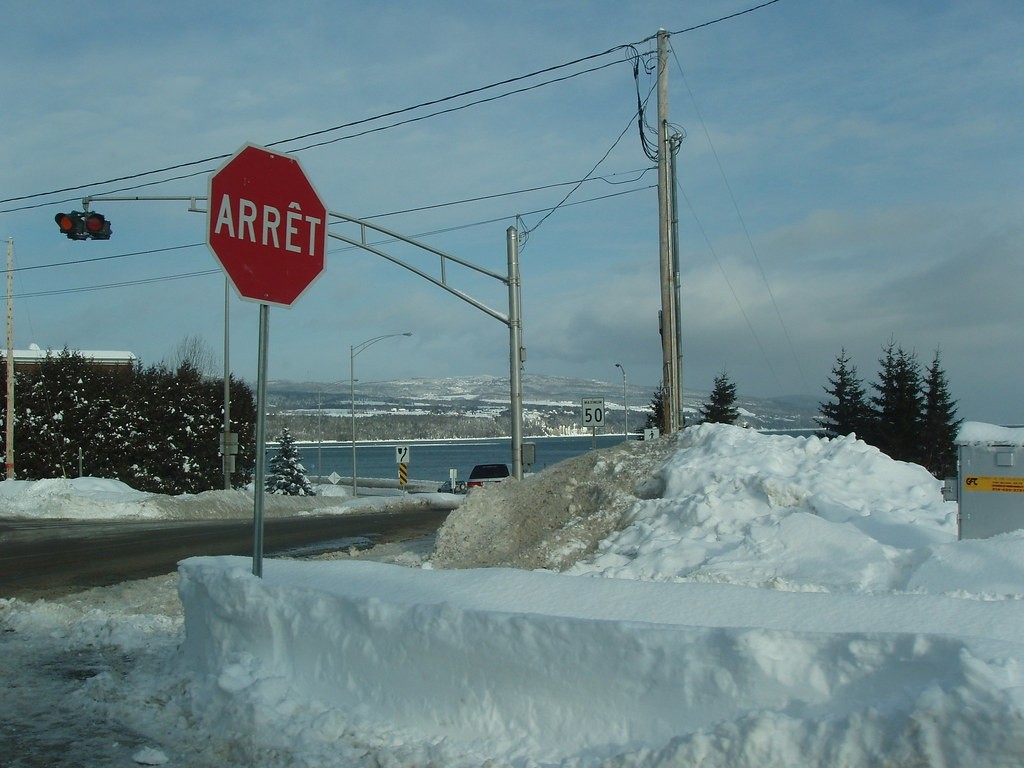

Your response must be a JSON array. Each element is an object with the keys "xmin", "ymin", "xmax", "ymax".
[
  {"xmin": 54, "ymin": 194, "xmax": 231, "ymax": 490},
  {"xmin": 615, "ymin": 363, "xmax": 629, "ymax": 441},
  {"xmin": 350, "ymin": 330, "xmax": 413, "ymax": 497}
]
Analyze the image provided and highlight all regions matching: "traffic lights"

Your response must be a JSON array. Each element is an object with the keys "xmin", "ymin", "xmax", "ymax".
[{"xmin": 55, "ymin": 208, "xmax": 114, "ymax": 240}]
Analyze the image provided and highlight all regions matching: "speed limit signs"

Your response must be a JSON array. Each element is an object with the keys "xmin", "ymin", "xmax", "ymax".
[{"xmin": 582, "ymin": 397, "xmax": 605, "ymax": 427}]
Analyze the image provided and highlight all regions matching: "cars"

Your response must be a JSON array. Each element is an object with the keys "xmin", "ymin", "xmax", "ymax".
[
  {"xmin": 467, "ymin": 463, "xmax": 513, "ymax": 492},
  {"xmin": 438, "ymin": 479, "xmax": 467, "ymax": 496}
]
